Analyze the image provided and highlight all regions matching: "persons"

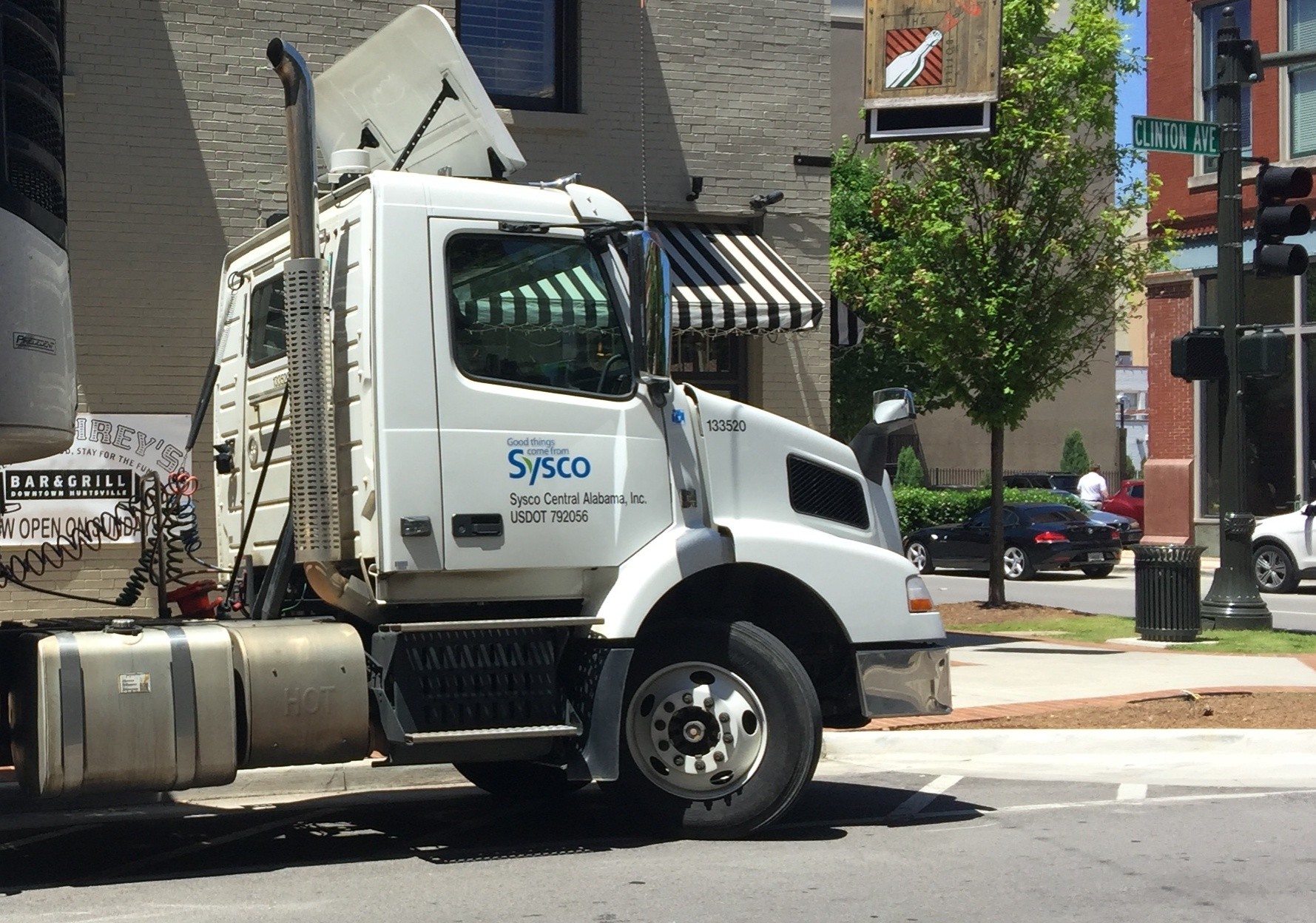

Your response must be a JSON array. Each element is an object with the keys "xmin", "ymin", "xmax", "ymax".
[{"xmin": 1077, "ymin": 464, "xmax": 1109, "ymax": 510}]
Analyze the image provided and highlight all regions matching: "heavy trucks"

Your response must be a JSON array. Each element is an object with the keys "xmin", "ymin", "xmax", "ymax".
[{"xmin": 2, "ymin": 3, "xmax": 949, "ymax": 840}]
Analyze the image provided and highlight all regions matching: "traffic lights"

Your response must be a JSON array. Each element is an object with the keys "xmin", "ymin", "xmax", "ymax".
[
  {"xmin": 1171, "ymin": 335, "xmax": 1225, "ymax": 384},
  {"xmin": 1254, "ymin": 164, "xmax": 1312, "ymax": 279}
]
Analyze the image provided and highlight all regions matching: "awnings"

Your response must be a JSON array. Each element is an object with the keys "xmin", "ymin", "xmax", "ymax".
[{"xmin": 454, "ymin": 224, "xmax": 826, "ymax": 336}]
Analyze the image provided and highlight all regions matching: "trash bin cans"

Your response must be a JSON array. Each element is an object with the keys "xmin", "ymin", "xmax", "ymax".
[{"xmin": 1127, "ymin": 543, "xmax": 1208, "ymax": 642}]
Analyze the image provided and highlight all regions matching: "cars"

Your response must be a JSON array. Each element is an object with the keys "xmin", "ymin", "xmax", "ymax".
[
  {"xmin": 902, "ymin": 502, "xmax": 1122, "ymax": 582},
  {"xmin": 1251, "ymin": 500, "xmax": 1316, "ymax": 593},
  {"xmin": 986, "ymin": 473, "xmax": 1143, "ymax": 544}
]
